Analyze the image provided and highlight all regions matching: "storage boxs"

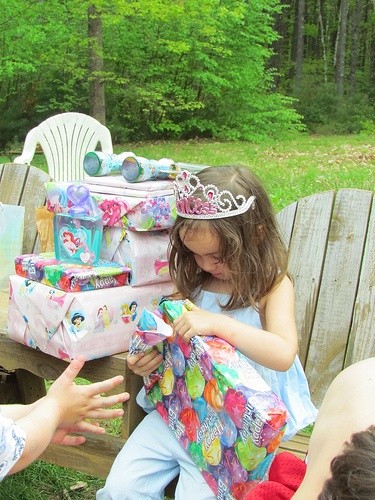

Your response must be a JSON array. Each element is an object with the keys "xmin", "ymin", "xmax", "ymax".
[
  {"xmin": 5, "ymin": 182, "xmax": 191, "ymax": 363},
  {"xmin": 129, "ymin": 298, "xmax": 289, "ymax": 500}
]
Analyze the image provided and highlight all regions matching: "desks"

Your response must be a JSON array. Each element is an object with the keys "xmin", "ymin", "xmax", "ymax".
[{"xmin": 0, "ymin": 288, "xmax": 155, "ymax": 480}]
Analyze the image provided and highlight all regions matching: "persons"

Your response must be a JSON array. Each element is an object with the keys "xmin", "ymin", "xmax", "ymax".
[
  {"xmin": 290, "ymin": 356, "xmax": 375, "ymax": 500},
  {"xmin": 0, "ymin": 356, "xmax": 130, "ymax": 483},
  {"xmin": 96, "ymin": 165, "xmax": 318, "ymax": 500}
]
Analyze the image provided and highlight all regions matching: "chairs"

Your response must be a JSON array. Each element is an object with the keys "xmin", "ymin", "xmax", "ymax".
[
  {"xmin": 272, "ymin": 187, "xmax": 375, "ymax": 462},
  {"xmin": 0, "ymin": 162, "xmax": 53, "ymax": 254},
  {"xmin": 15, "ymin": 112, "xmax": 113, "ymax": 181}
]
